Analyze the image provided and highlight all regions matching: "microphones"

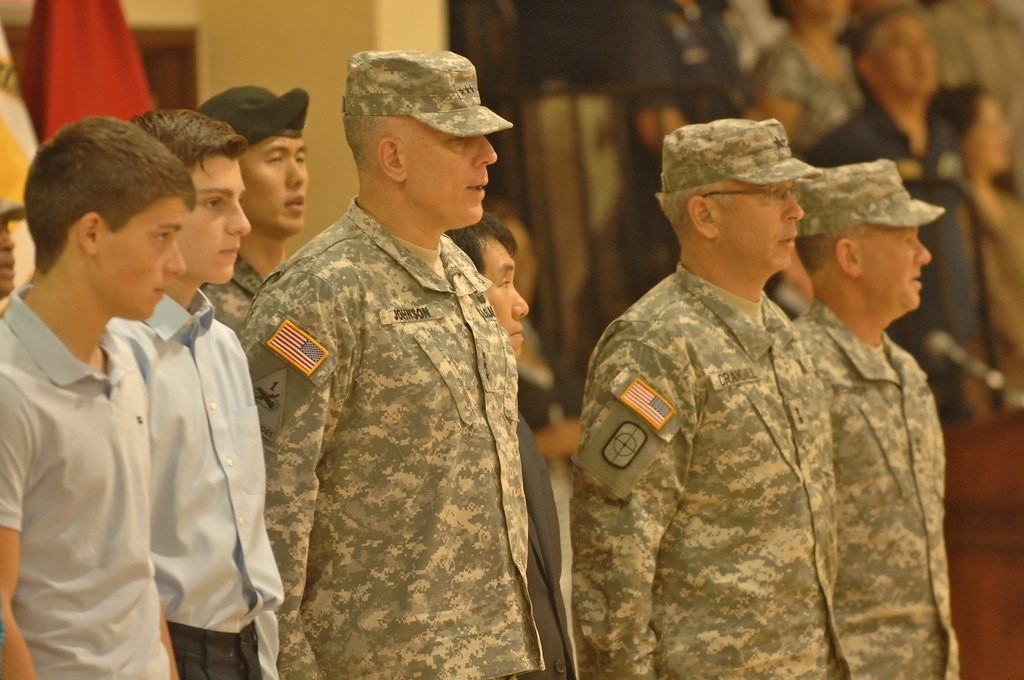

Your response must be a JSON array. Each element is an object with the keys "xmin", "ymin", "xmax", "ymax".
[{"xmin": 925, "ymin": 330, "xmax": 1024, "ymax": 409}]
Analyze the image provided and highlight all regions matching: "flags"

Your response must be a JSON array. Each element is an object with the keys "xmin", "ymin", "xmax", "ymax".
[{"xmin": 0, "ymin": 1, "xmax": 157, "ymax": 305}]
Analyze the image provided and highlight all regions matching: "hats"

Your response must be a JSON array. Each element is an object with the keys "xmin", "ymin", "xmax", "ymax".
[
  {"xmin": 0, "ymin": 197, "xmax": 28, "ymax": 220},
  {"xmin": 195, "ymin": 85, "xmax": 309, "ymax": 145},
  {"xmin": 797, "ymin": 158, "xmax": 945, "ymax": 236},
  {"xmin": 343, "ymin": 50, "xmax": 513, "ymax": 136},
  {"xmin": 660, "ymin": 118, "xmax": 822, "ymax": 185}
]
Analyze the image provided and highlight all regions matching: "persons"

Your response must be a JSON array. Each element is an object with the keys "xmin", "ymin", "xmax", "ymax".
[
  {"xmin": 0, "ymin": 113, "xmax": 177, "ymax": 680},
  {"xmin": 448, "ymin": 0, "xmax": 1023, "ymax": 424},
  {"xmin": 571, "ymin": 117, "xmax": 856, "ymax": 679},
  {"xmin": 237, "ymin": 48, "xmax": 546, "ymax": 680},
  {"xmin": 444, "ymin": 210, "xmax": 578, "ymax": 680},
  {"xmin": 790, "ymin": 157, "xmax": 962, "ymax": 680},
  {"xmin": 0, "ymin": 196, "xmax": 16, "ymax": 304},
  {"xmin": 197, "ymin": 83, "xmax": 309, "ymax": 342},
  {"xmin": 127, "ymin": 109, "xmax": 284, "ymax": 680}
]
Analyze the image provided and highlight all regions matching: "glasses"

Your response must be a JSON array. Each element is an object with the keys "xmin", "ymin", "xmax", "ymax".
[{"xmin": 703, "ymin": 185, "xmax": 801, "ymax": 210}]
